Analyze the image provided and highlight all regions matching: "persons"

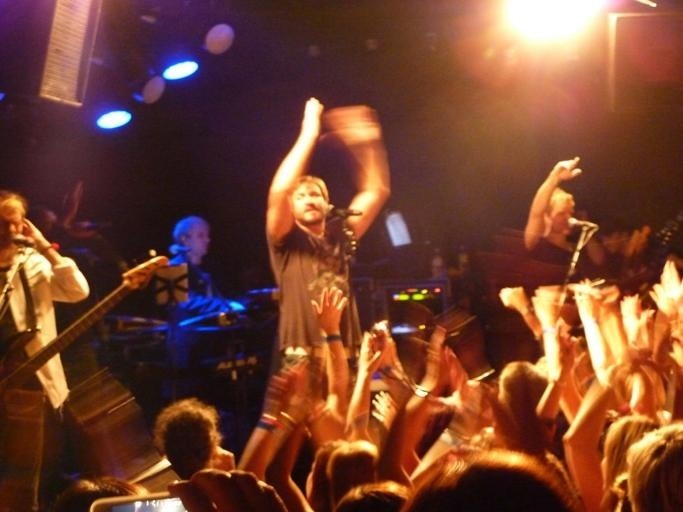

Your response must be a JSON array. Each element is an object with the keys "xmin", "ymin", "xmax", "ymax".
[
  {"xmin": 25, "ymin": 177, "xmax": 97, "ymax": 254},
  {"xmin": 152, "ymin": 215, "xmax": 245, "ymax": 404},
  {"xmin": 51, "ymin": 256, "xmax": 682, "ymax": 511},
  {"xmin": 0, "ymin": 186, "xmax": 91, "ymax": 512},
  {"xmin": 523, "ymin": 156, "xmax": 609, "ymax": 291},
  {"xmin": 261, "ymin": 96, "xmax": 392, "ymax": 383}
]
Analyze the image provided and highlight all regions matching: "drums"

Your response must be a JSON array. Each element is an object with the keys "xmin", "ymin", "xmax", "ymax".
[
  {"xmin": 103, "ymin": 313, "xmax": 173, "ymax": 388},
  {"xmin": 247, "ymin": 287, "xmax": 281, "ymax": 351},
  {"xmin": 201, "ymin": 355, "xmax": 266, "ymax": 409}
]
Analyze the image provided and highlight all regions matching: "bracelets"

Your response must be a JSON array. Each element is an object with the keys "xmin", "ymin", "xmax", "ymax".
[
  {"xmin": 440, "ymin": 429, "xmax": 468, "ymax": 447},
  {"xmin": 279, "ymin": 411, "xmax": 299, "ymax": 431},
  {"xmin": 414, "ymin": 385, "xmax": 433, "ymax": 398},
  {"xmin": 256, "ymin": 413, "xmax": 279, "ymax": 431},
  {"xmin": 325, "ymin": 332, "xmax": 342, "ymax": 342}
]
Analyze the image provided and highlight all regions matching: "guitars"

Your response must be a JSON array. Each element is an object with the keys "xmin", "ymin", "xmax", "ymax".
[{"xmin": 0, "ymin": 248, "xmax": 171, "ymax": 400}]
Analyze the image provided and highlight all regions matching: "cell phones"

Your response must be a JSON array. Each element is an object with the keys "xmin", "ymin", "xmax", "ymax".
[{"xmin": 90, "ymin": 491, "xmax": 190, "ymax": 512}]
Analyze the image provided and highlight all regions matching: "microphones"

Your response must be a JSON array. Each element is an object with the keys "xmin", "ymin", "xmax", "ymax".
[
  {"xmin": 567, "ymin": 217, "xmax": 597, "ymax": 228},
  {"xmin": 169, "ymin": 245, "xmax": 193, "ymax": 255},
  {"xmin": 13, "ymin": 233, "xmax": 36, "ymax": 247},
  {"xmin": 325, "ymin": 203, "xmax": 362, "ymax": 217}
]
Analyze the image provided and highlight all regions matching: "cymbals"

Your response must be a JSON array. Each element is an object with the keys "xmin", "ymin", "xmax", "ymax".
[{"xmin": 177, "ymin": 300, "xmax": 279, "ymax": 335}]
